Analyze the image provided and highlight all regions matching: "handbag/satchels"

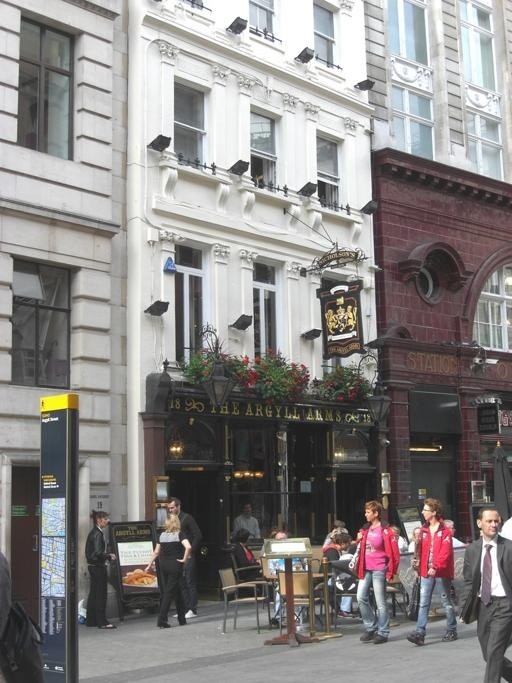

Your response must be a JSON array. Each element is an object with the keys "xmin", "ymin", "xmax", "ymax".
[{"xmin": 405, "ymin": 579, "xmax": 421, "ymax": 622}]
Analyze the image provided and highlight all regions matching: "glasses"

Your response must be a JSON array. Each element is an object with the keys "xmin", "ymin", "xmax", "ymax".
[{"xmin": 423, "ymin": 508, "xmax": 432, "ymax": 512}]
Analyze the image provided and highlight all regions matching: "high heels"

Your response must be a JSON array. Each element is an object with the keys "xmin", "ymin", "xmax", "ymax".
[
  {"xmin": 157, "ymin": 622, "xmax": 170, "ymax": 630},
  {"xmin": 98, "ymin": 623, "xmax": 118, "ymax": 629}
]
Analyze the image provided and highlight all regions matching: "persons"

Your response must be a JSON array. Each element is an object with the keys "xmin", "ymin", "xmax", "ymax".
[
  {"xmin": 458, "ymin": 507, "xmax": 512, "ymax": 683},
  {"xmin": 0, "ymin": 553, "xmax": 44, "ymax": 683},
  {"xmin": 234, "ymin": 528, "xmax": 289, "ymax": 627},
  {"xmin": 268, "ymin": 530, "xmax": 309, "ymax": 629},
  {"xmin": 232, "ymin": 504, "xmax": 261, "ymax": 539},
  {"xmin": 443, "ymin": 519, "xmax": 465, "ymax": 548},
  {"xmin": 86, "ymin": 510, "xmax": 117, "ymax": 629},
  {"xmin": 167, "ymin": 497, "xmax": 203, "ymax": 620},
  {"xmin": 356, "ymin": 500, "xmax": 400, "ymax": 644},
  {"xmin": 313, "ymin": 519, "xmax": 371, "ymax": 617},
  {"xmin": 407, "ymin": 528, "xmax": 422, "ymax": 553},
  {"xmin": 405, "ymin": 497, "xmax": 457, "ymax": 646},
  {"xmin": 144, "ymin": 514, "xmax": 192, "ymax": 627},
  {"xmin": 389, "ymin": 524, "xmax": 407, "ymax": 554}
]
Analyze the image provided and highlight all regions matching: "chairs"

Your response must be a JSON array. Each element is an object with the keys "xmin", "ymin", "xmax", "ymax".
[{"xmin": 216, "ymin": 545, "xmax": 408, "ymax": 640}]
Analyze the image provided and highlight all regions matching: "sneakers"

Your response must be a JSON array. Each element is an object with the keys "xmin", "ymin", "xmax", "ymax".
[
  {"xmin": 374, "ymin": 634, "xmax": 388, "ymax": 644},
  {"xmin": 407, "ymin": 630, "xmax": 425, "ymax": 646},
  {"xmin": 360, "ymin": 631, "xmax": 377, "ymax": 641},
  {"xmin": 442, "ymin": 629, "xmax": 458, "ymax": 642},
  {"xmin": 173, "ymin": 609, "xmax": 199, "ymax": 619},
  {"xmin": 459, "ymin": 591, "xmax": 481, "ymax": 624}
]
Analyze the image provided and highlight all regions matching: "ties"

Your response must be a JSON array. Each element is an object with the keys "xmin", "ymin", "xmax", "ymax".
[{"xmin": 481, "ymin": 544, "xmax": 493, "ymax": 607}]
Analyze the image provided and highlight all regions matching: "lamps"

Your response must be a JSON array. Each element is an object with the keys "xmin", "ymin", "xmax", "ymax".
[
  {"xmin": 197, "ymin": 329, "xmax": 237, "ymax": 414},
  {"xmin": 148, "ymin": 134, "xmax": 171, "ymax": 153},
  {"xmin": 355, "ymin": 79, "xmax": 375, "ymax": 91},
  {"xmin": 299, "ymin": 328, "xmax": 323, "ymax": 341},
  {"xmin": 357, "ymin": 356, "xmax": 393, "ymax": 426},
  {"xmin": 361, "ymin": 198, "xmax": 381, "ymax": 216},
  {"xmin": 296, "ymin": 47, "xmax": 316, "ymax": 65},
  {"xmin": 228, "ymin": 160, "xmax": 250, "ymax": 175},
  {"xmin": 230, "ymin": 313, "xmax": 253, "ymax": 333},
  {"xmin": 227, "ymin": 16, "xmax": 249, "ymax": 34},
  {"xmin": 298, "ymin": 181, "xmax": 317, "ymax": 196},
  {"xmin": 146, "ymin": 302, "xmax": 170, "ymax": 318}
]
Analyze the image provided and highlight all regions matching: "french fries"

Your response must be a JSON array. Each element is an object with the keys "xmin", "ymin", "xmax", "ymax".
[{"xmin": 127, "ymin": 572, "xmax": 155, "ymax": 584}]
